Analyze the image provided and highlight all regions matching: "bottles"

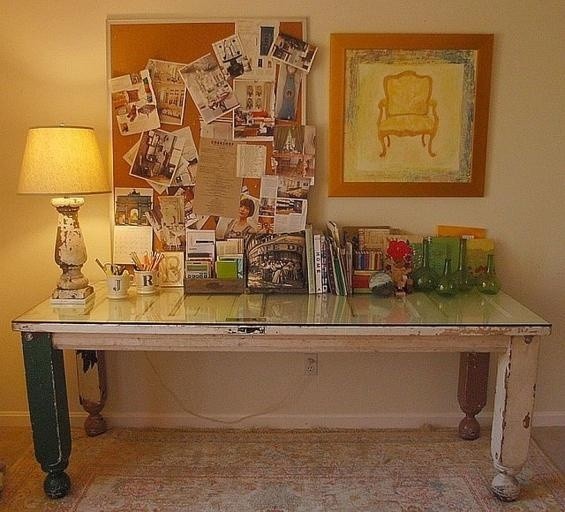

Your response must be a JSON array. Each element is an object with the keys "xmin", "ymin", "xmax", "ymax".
[{"xmin": 411, "ymin": 237, "xmax": 501, "ymax": 298}]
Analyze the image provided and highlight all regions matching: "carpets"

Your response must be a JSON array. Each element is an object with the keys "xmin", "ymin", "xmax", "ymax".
[{"xmin": 0, "ymin": 428, "xmax": 565, "ymax": 511}]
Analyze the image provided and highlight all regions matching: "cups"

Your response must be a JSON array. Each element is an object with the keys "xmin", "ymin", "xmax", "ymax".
[
  {"xmin": 134, "ymin": 271, "xmax": 162, "ymax": 295},
  {"xmin": 106, "ymin": 275, "xmax": 135, "ymax": 299}
]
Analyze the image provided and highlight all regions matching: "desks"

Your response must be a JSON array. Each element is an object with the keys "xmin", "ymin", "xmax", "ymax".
[{"xmin": 10, "ymin": 284, "xmax": 553, "ymax": 501}]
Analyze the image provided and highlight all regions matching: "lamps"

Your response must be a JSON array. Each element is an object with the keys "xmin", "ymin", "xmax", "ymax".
[{"xmin": 16, "ymin": 123, "xmax": 112, "ymax": 310}]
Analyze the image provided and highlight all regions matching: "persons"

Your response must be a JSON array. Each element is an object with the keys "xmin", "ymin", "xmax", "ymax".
[
  {"xmin": 257, "ymin": 259, "xmax": 304, "ymax": 285},
  {"xmin": 222, "ymin": 198, "xmax": 256, "ymax": 242}
]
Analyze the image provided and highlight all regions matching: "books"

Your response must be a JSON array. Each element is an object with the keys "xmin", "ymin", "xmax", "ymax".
[{"xmin": 305, "ymin": 219, "xmax": 353, "ymax": 296}]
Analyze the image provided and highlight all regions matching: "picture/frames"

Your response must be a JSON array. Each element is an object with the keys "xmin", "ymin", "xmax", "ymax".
[{"xmin": 328, "ymin": 32, "xmax": 494, "ymax": 198}]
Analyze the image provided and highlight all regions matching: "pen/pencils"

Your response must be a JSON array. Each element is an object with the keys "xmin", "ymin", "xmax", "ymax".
[
  {"xmin": 95, "ymin": 258, "xmax": 126, "ymax": 275},
  {"xmin": 129, "ymin": 250, "xmax": 164, "ymax": 271}
]
